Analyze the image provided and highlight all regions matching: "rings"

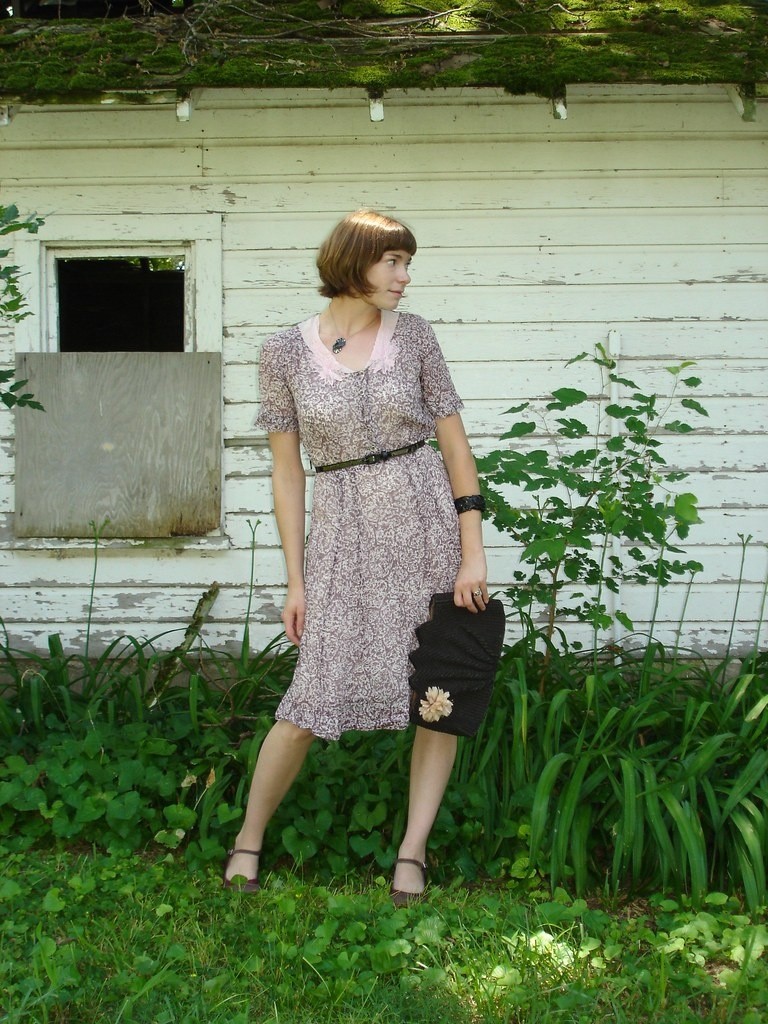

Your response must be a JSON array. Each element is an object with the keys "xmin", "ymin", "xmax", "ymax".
[{"xmin": 473, "ymin": 590, "xmax": 480, "ymax": 596}]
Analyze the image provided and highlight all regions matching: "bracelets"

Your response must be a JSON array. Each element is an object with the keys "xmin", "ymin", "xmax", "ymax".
[{"xmin": 454, "ymin": 495, "xmax": 486, "ymax": 514}]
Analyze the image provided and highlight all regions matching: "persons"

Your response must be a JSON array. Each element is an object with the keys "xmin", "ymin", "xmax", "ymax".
[{"xmin": 222, "ymin": 210, "xmax": 490, "ymax": 907}]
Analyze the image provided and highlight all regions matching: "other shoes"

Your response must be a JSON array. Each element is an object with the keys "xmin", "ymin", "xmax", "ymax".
[
  {"xmin": 390, "ymin": 857, "xmax": 427, "ymax": 908},
  {"xmin": 224, "ymin": 846, "xmax": 261, "ymax": 893}
]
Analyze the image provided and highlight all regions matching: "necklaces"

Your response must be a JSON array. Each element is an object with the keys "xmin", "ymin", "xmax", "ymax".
[{"xmin": 328, "ymin": 303, "xmax": 380, "ymax": 354}]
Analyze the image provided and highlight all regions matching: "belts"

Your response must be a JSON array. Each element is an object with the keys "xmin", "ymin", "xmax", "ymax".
[{"xmin": 315, "ymin": 439, "xmax": 425, "ymax": 473}]
